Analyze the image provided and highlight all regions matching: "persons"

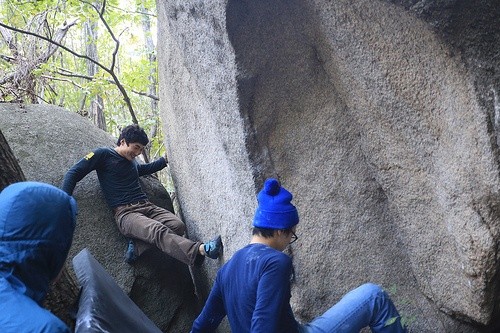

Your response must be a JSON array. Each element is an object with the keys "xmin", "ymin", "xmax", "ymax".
[
  {"xmin": 0, "ymin": 180, "xmax": 77, "ymax": 333},
  {"xmin": 62, "ymin": 124, "xmax": 222, "ymax": 263},
  {"xmin": 191, "ymin": 178, "xmax": 403, "ymax": 332}
]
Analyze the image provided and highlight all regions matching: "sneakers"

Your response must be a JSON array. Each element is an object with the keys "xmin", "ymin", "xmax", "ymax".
[
  {"xmin": 203, "ymin": 235, "xmax": 222, "ymax": 259},
  {"xmin": 124, "ymin": 241, "xmax": 138, "ymax": 264}
]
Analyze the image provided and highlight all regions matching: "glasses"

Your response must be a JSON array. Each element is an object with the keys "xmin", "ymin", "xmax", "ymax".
[{"xmin": 287, "ymin": 228, "xmax": 298, "ymax": 244}]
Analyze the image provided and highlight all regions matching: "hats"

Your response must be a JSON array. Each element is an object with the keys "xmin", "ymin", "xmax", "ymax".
[{"xmin": 252, "ymin": 177, "xmax": 299, "ymax": 229}]
